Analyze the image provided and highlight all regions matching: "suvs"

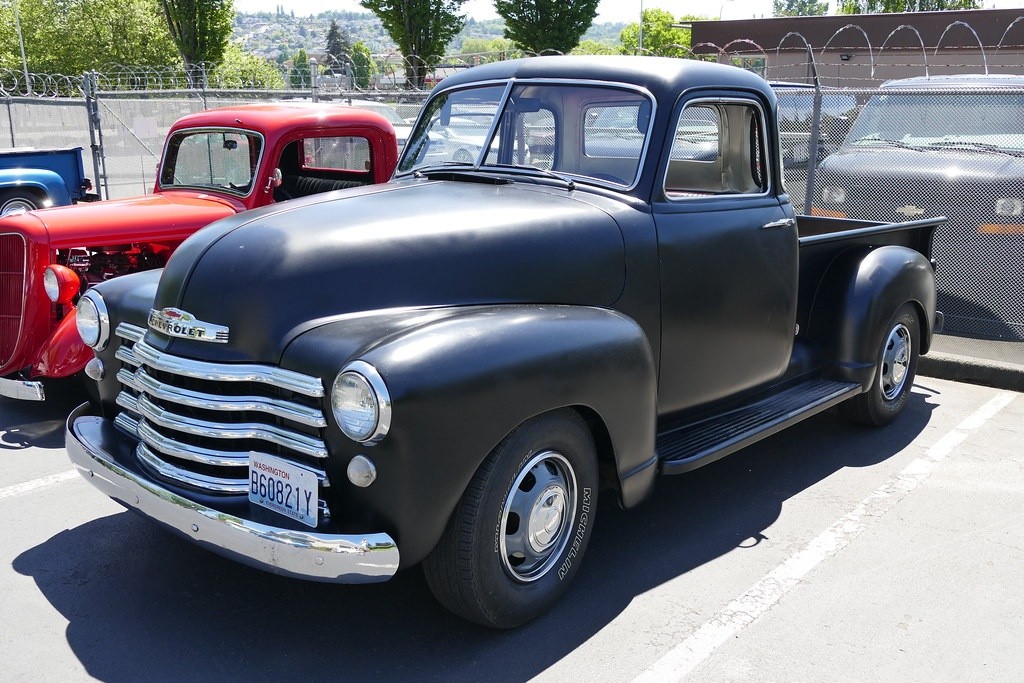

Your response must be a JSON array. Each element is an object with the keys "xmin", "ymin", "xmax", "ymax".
[{"xmin": 316, "ymin": 62, "xmax": 377, "ymax": 91}]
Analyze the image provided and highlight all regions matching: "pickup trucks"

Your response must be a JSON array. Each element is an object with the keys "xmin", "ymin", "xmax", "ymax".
[
  {"xmin": 0, "ymin": 146, "xmax": 92, "ymax": 220},
  {"xmin": 65, "ymin": 54, "xmax": 948, "ymax": 641},
  {"xmin": 0, "ymin": 102, "xmax": 398, "ymax": 424}
]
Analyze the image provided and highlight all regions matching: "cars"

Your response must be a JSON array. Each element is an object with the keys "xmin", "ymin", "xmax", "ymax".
[
  {"xmin": 808, "ymin": 74, "xmax": 1024, "ymax": 341},
  {"xmin": 545, "ymin": 79, "xmax": 858, "ymax": 219},
  {"xmin": 296, "ymin": 90, "xmax": 600, "ymax": 178}
]
